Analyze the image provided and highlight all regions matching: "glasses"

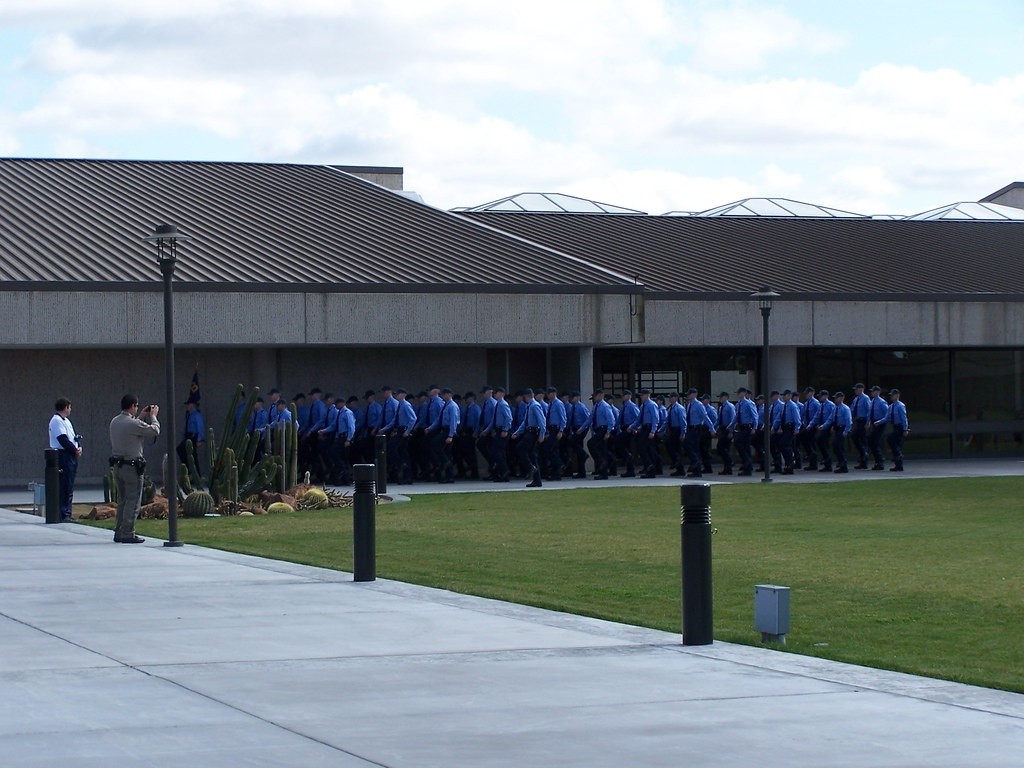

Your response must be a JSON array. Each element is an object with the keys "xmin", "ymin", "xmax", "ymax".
[{"xmin": 135, "ymin": 405, "xmax": 139, "ymax": 407}]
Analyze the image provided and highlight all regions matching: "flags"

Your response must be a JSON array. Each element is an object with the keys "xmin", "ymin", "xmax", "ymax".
[{"xmin": 185, "ymin": 371, "xmax": 201, "ymax": 421}]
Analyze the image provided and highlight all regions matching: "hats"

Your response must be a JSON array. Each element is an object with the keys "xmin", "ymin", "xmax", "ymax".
[
  {"xmin": 184, "ymin": 396, "xmax": 200, "ymax": 406},
  {"xmin": 237, "ymin": 383, "xmax": 904, "ymax": 404}
]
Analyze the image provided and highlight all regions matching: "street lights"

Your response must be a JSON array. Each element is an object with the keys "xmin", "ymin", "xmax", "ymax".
[
  {"xmin": 141, "ymin": 224, "xmax": 193, "ymax": 549},
  {"xmin": 749, "ymin": 288, "xmax": 781, "ymax": 482}
]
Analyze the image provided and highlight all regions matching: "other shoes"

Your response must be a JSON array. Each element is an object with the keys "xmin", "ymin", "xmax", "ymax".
[
  {"xmin": 374, "ymin": 460, "xmax": 903, "ymax": 487},
  {"xmin": 114, "ymin": 536, "xmax": 145, "ymax": 543},
  {"xmin": 63, "ymin": 516, "xmax": 76, "ymax": 522}
]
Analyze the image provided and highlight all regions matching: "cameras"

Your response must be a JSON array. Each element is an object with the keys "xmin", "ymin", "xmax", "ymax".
[
  {"xmin": 74, "ymin": 434, "xmax": 83, "ymax": 442},
  {"xmin": 146, "ymin": 405, "xmax": 159, "ymax": 413}
]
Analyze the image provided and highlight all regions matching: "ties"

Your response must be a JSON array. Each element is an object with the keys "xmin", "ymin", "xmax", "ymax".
[
  {"xmin": 185, "ymin": 414, "xmax": 190, "ymax": 433},
  {"xmin": 246, "ymin": 397, "xmax": 896, "ymax": 434}
]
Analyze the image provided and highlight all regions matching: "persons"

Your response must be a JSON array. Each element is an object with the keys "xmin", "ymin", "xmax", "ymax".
[
  {"xmin": 48, "ymin": 399, "xmax": 83, "ymax": 523},
  {"xmin": 176, "ymin": 399, "xmax": 205, "ymax": 477},
  {"xmin": 109, "ymin": 394, "xmax": 160, "ymax": 543},
  {"xmin": 231, "ymin": 387, "xmax": 908, "ymax": 487}
]
